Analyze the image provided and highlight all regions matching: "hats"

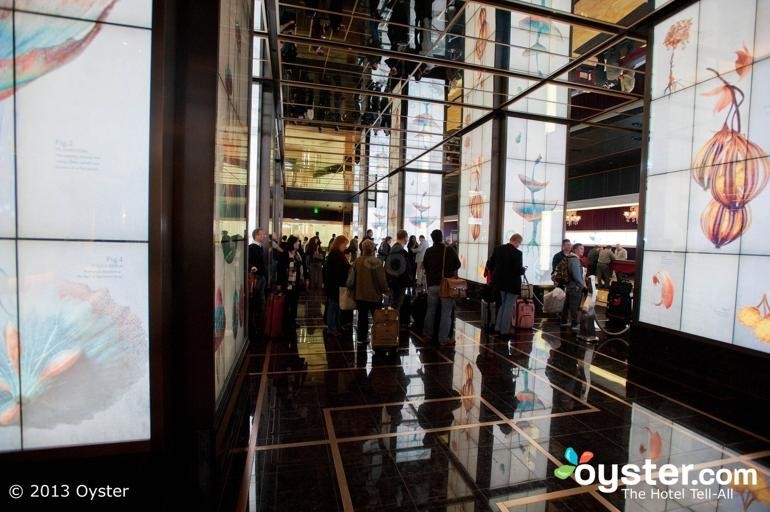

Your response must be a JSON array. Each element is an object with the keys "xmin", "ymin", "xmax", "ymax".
[
  {"xmin": 361, "ymin": 239, "xmax": 378, "ymax": 254},
  {"xmin": 385, "ymin": 253, "xmax": 406, "ymax": 276}
]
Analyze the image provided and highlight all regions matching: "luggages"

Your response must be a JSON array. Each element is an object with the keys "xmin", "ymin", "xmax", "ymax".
[
  {"xmin": 577, "ymin": 309, "xmax": 595, "ymax": 338},
  {"xmin": 512, "ymin": 298, "xmax": 535, "ymax": 328},
  {"xmin": 481, "ymin": 298, "xmax": 497, "ymax": 333},
  {"xmin": 410, "ymin": 293, "xmax": 455, "ymax": 336},
  {"xmin": 607, "ymin": 280, "xmax": 632, "ymax": 322},
  {"xmin": 371, "ymin": 306, "xmax": 400, "ymax": 349},
  {"xmin": 262, "ymin": 291, "xmax": 285, "ymax": 338}
]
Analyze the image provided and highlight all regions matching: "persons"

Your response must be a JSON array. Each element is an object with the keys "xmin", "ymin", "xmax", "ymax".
[
  {"xmin": 483, "ymin": 234, "xmax": 627, "ymax": 342},
  {"xmin": 248, "ymin": 228, "xmax": 460, "ymax": 343}
]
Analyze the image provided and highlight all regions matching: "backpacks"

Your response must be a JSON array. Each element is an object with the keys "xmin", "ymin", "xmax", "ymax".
[{"xmin": 554, "ymin": 257, "xmax": 570, "ymax": 284}]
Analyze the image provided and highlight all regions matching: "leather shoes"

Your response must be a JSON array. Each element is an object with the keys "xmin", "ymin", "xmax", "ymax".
[{"xmin": 326, "ymin": 327, "xmax": 342, "ymax": 337}]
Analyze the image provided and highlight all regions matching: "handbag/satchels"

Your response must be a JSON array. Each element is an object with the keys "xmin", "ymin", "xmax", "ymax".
[
  {"xmin": 439, "ymin": 277, "xmax": 467, "ymax": 299},
  {"xmin": 384, "ymin": 294, "xmax": 411, "ymax": 320},
  {"xmin": 247, "ymin": 269, "xmax": 257, "ymax": 297},
  {"xmin": 338, "ymin": 286, "xmax": 358, "ymax": 311},
  {"xmin": 346, "ymin": 268, "xmax": 355, "ymax": 287}
]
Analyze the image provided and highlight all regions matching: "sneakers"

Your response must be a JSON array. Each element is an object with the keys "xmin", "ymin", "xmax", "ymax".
[
  {"xmin": 440, "ymin": 339, "xmax": 455, "ymax": 345},
  {"xmin": 357, "ymin": 339, "xmax": 369, "ymax": 343},
  {"xmin": 499, "ymin": 333, "xmax": 517, "ymax": 338},
  {"xmin": 560, "ymin": 322, "xmax": 580, "ymax": 330}
]
